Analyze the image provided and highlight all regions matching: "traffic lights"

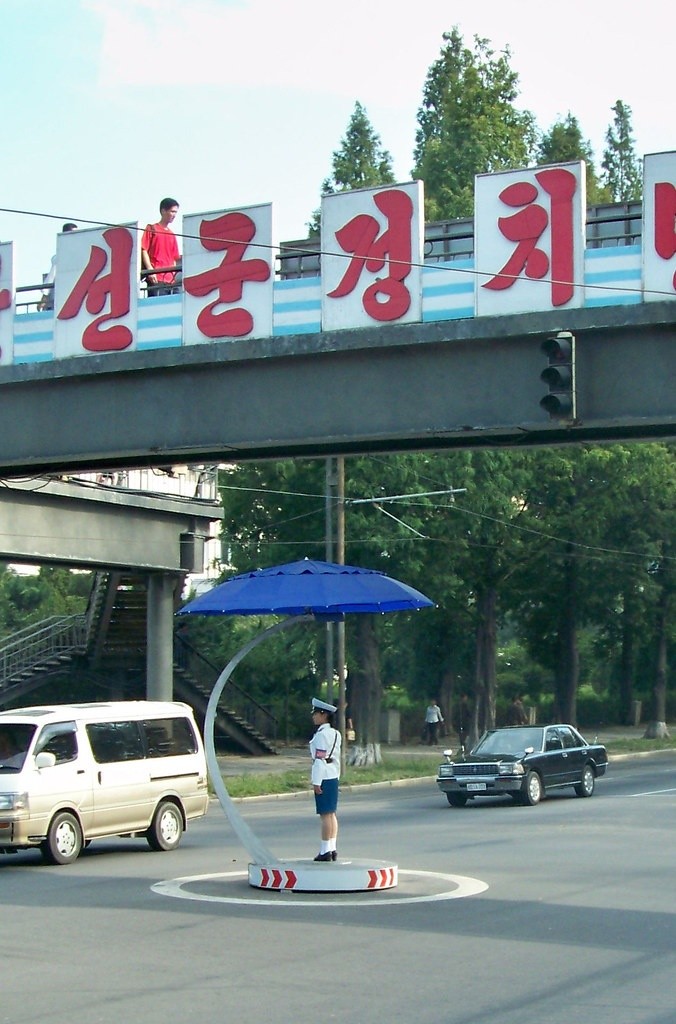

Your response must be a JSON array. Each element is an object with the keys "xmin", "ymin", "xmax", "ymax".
[{"xmin": 540, "ymin": 332, "xmax": 579, "ymax": 422}]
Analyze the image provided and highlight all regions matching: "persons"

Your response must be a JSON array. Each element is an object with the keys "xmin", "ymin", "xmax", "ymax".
[
  {"xmin": 332, "ymin": 702, "xmax": 352, "ymax": 729},
  {"xmin": 453, "ymin": 693, "xmax": 470, "ymax": 746},
  {"xmin": 509, "ymin": 696, "xmax": 527, "ymax": 725},
  {"xmin": 37, "ymin": 223, "xmax": 78, "ymax": 311},
  {"xmin": 309, "ymin": 698, "xmax": 342, "ymax": 862},
  {"xmin": 425, "ymin": 699, "xmax": 444, "ymax": 746},
  {"xmin": 141, "ymin": 198, "xmax": 179, "ymax": 296}
]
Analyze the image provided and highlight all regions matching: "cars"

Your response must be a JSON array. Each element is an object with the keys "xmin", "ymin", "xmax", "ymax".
[{"xmin": 436, "ymin": 723, "xmax": 610, "ymax": 807}]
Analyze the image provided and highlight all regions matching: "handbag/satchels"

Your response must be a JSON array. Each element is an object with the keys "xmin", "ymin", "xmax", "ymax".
[{"xmin": 421, "ymin": 726, "xmax": 428, "ymax": 741}]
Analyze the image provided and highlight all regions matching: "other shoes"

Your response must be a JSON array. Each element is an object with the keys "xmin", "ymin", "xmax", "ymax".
[
  {"xmin": 435, "ymin": 740, "xmax": 438, "ymax": 744},
  {"xmin": 313, "ymin": 851, "xmax": 331, "ymax": 860},
  {"xmin": 331, "ymin": 851, "xmax": 337, "ymax": 861},
  {"xmin": 429, "ymin": 742, "xmax": 433, "ymax": 746}
]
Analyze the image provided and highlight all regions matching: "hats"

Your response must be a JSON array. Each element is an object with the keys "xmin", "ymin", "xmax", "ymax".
[{"xmin": 310, "ymin": 697, "xmax": 338, "ymax": 714}]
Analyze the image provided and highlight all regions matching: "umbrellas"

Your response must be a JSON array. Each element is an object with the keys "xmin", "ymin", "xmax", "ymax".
[{"xmin": 175, "ymin": 556, "xmax": 436, "ymax": 866}]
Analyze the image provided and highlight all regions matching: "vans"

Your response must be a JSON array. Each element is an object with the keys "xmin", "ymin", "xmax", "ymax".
[{"xmin": 0, "ymin": 701, "xmax": 208, "ymax": 866}]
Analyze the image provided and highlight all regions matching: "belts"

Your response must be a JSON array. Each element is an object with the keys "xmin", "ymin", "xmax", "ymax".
[{"xmin": 311, "ymin": 759, "xmax": 333, "ymax": 763}]
